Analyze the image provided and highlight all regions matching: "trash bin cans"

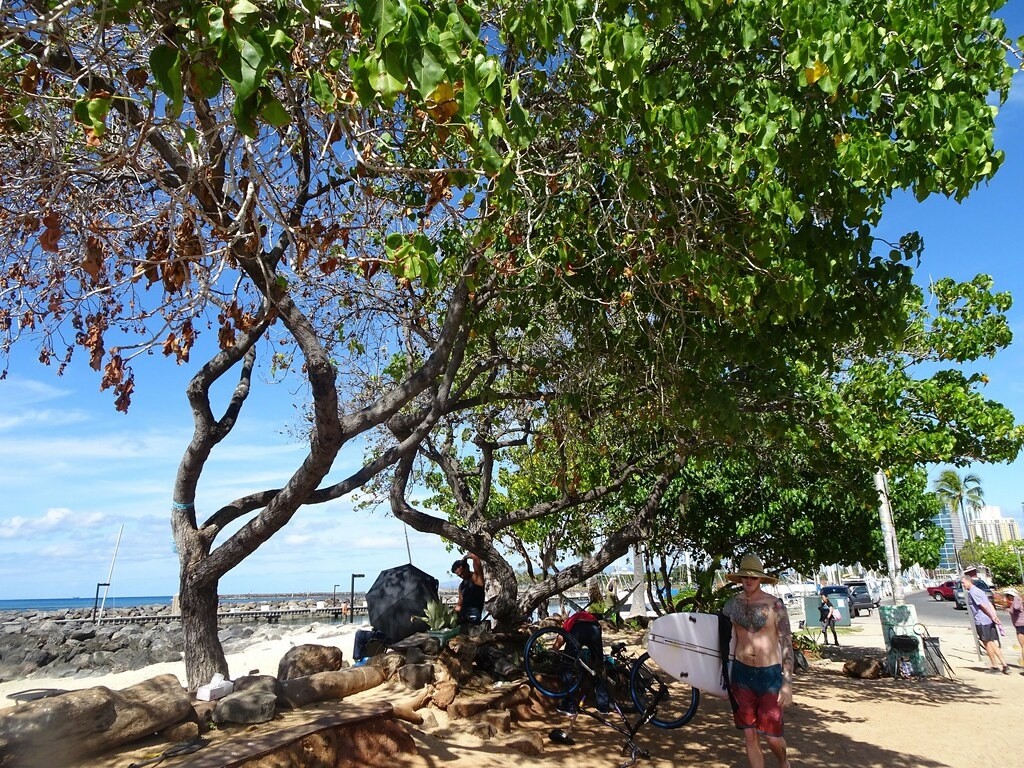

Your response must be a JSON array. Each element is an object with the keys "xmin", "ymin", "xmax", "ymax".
[{"xmin": 803, "ymin": 595, "xmax": 852, "ymax": 627}]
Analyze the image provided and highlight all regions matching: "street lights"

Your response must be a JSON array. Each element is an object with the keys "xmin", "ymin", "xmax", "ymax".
[
  {"xmin": 93, "ymin": 583, "xmax": 109, "ymax": 624},
  {"xmin": 350, "ymin": 573, "xmax": 365, "ymax": 623},
  {"xmin": 333, "ymin": 584, "xmax": 340, "ymax": 605}
]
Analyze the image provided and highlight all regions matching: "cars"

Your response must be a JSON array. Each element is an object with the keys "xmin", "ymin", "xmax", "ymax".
[{"xmin": 820, "ymin": 586, "xmax": 855, "ymax": 617}]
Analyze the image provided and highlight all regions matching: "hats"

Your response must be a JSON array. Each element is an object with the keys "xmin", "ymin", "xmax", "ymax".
[
  {"xmin": 1003, "ymin": 588, "xmax": 1018, "ymax": 597},
  {"xmin": 964, "ymin": 565, "xmax": 976, "ymax": 575},
  {"xmin": 725, "ymin": 555, "xmax": 776, "ymax": 584}
]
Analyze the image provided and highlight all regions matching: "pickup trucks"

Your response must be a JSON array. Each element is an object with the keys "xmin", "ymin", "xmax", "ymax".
[{"xmin": 927, "ymin": 579, "xmax": 958, "ymax": 601}]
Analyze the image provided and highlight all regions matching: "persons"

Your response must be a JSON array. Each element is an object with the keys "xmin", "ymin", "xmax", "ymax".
[
  {"xmin": 817, "ymin": 593, "xmax": 839, "ymax": 646},
  {"xmin": 1002, "ymin": 589, "xmax": 1024, "ymax": 668},
  {"xmin": 341, "ymin": 601, "xmax": 347, "ymax": 625},
  {"xmin": 553, "ymin": 613, "xmax": 610, "ymax": 716},
  {"xmin": 452, "ymin": 552, "xmax": 485, "ymax": 631},
  {"xmin": 963, "ymin": 565, "xmax": 1001, "ymax": 648},
  {"xmin": 719, "ymin": 554, "xmax": 794, "ymax": 768},
  {"xmin": 960, "ymin": 575, "xmax": 1012, "ymax": 674}
]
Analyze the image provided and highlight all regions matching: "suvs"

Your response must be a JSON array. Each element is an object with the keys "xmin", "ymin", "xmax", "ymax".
[
  {"xmin": 843, "ymin": 578, "xmax": 880, "ymax": 607},
  {"xmin": 950, "ymin": 579, "xmax": 996, "ymax": 610}
]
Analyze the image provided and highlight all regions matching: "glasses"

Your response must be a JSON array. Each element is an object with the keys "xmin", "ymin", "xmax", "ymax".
[{"xmin": 740, "ymin": 575, "xmax": 759, "ymax": 580}]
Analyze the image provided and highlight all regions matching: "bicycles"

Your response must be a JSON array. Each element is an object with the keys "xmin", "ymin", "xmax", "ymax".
[{"xmin": 522, "ymin": 626, "xmax": 698, "ymax": 768}]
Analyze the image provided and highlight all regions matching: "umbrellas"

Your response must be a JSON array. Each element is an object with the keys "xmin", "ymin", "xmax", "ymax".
[{"xmin": 364, "ymin": 564, "xmax": 439, "ymax": 648}]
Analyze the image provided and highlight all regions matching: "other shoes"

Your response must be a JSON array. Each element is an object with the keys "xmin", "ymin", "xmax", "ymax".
[
  {"xmin": 1003, "ymin": 666, "xmax": 1011, "ymax": 673},
  {"xmin": 596, "ymin": 706, "xmax": 608, "ymax": 714},
  {"xmin": 822, "ymin": 642, "xmax": 828, "ymax": 645},
  {"xmin": 984, "ymin": 667, "xmax": 1001, "ymax": 674},
  {"xmin": 555, "ymin": 705, "xmax": 576, "ymax": 716},
  {"xmin": 835, "ymin": 642, "xmax": 839, "ymax": 646}
]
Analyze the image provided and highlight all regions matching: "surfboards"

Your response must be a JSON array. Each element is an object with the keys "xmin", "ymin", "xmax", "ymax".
[{"xmin": 647, "ymin": 612, "xmax": 783, "ymax": 699}]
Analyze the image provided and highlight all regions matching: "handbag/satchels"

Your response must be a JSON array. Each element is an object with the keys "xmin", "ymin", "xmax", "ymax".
[{"xmin": 831, "ymin": 609, "xmax": 842, "ymax": 622}]
